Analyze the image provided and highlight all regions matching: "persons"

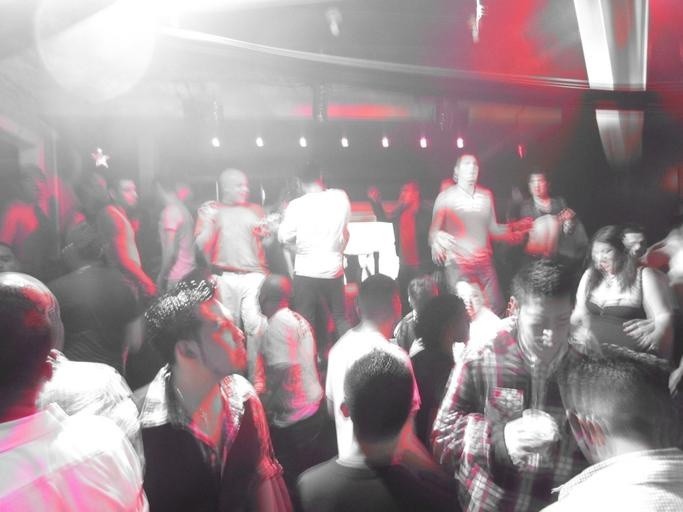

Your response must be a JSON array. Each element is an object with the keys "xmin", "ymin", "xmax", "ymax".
[
  {"xmin": 44, "ymin": 223, "xmax": 144, "ymax": 378},
  {"xmin": 569, "ymin": 224, "xmax": 674, "ymax": 361},
  {"xmin": 431, "ymin": 258, "xmax": 592, "ymax": 512},
  {"xmin": 20, "ymin": 272, "xmax": 145, "ymax": 480},
  {"xmin": 408, "ymin": 294, "xmax": 471, "ymax": 442},
  {"xmin": 2, "ymin": 244, "xmax": 20, "ymax": 272},
  {"xmin": 155, "ymin": 174, "xmax": 197, "ymax": 292},
  {"xmin": 288, "ymin": 351, "xmax": 457, "ymax": 512},
  {"xmin": 0, "ymin": 161, "xmax": 52, "ymax": 242},
  {"xmin": 622, "ymin": 224, "xmax": 646, "ymax": 259},
  {"xmin": 639, "ymin": 227, "xmax": 683, "ymax": 392},
  {"xmin": 0, "ymin": 272, "xmax": 150, "ymax": 512},
  {"xmin": 452, "ymin": 275, "xmax": 503, "ymax": 362},
  {"xmin": 98, "ymin": 176, "xmax": 157, "ymax": 297},
  {"xmin": 429, "ymin": 154, "xmax": 531, "ymax": 319},
  {"xmin": 194, "ymin": 167, "xmax": 267, "ymax": 385},
  {"xmin": 277, "ymin": 172, "xmax": 350, "ymax": 369},
  {"xmin": 508, "ymin": 166, "xmax": 588, "ymax": 260},
  {"xmin": 58, "ymin": 171, "xmax": 109, "ymax": 248},
  {"xmin": 326, "ymin": 274, "xmax": 450, "ymax": 484},
  {"xmin": 108, "ymin": 272, "xmax": 294, "ymax": 512},
  {"xmin": 556, "ymin": 357, "xmax": 683, "ymax": 512},
  {"xmin": 394, "ymin": 275, "xmax": 439, "ymax": 352},
  {"xmin": 367, "ymin": 180, "xmax": 434, "ymax": 316}
]
